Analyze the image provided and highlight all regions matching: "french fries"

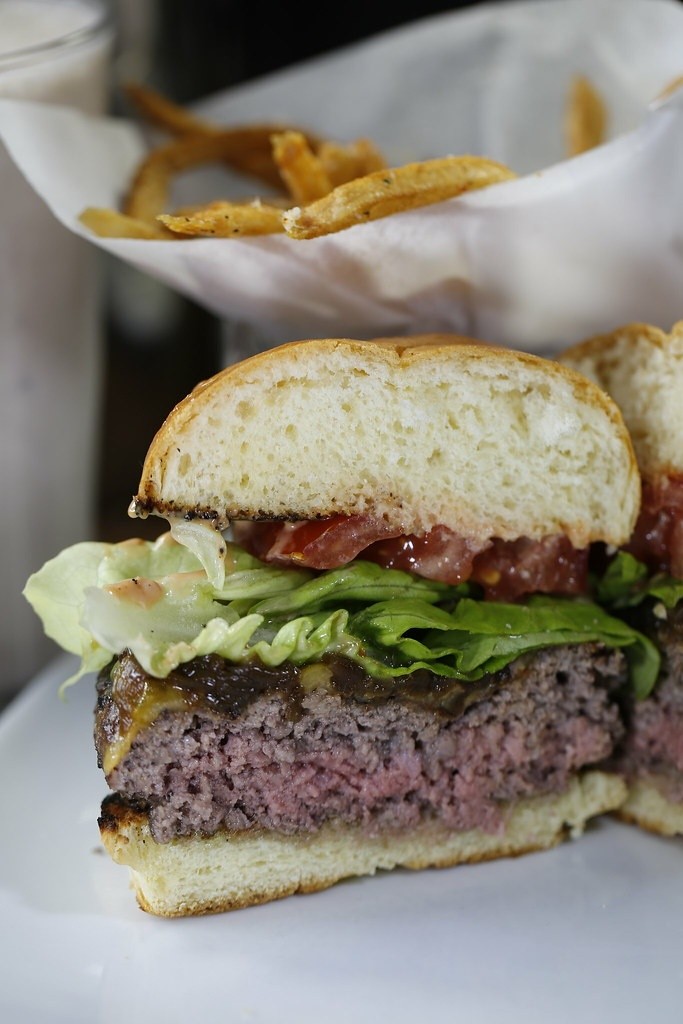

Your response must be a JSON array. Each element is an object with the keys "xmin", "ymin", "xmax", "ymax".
[{"xmin": 76, "ymin": 74, "xmax": 683, "ymax": 240}]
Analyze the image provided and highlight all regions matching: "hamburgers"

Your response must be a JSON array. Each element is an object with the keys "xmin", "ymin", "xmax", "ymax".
[{"xmin": 24, "ymin": 320, "xmax": 682, "ymax": 914}]
